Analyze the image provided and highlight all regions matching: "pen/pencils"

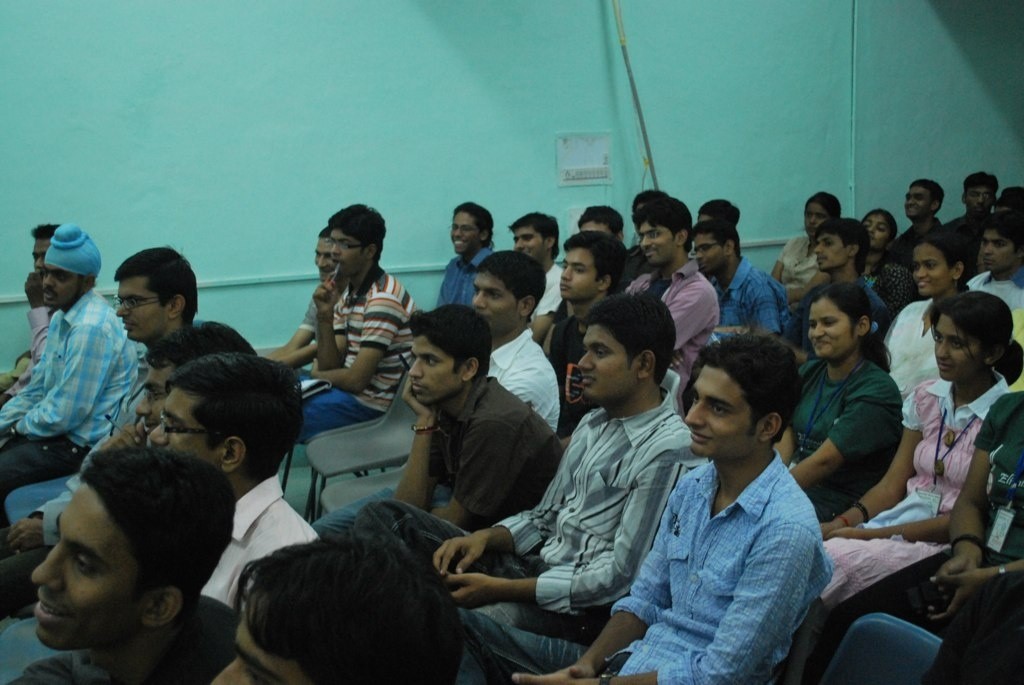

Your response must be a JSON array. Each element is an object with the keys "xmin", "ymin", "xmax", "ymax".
[
  {"xmin": 105, "ymin": 414, "xmax": 123, "ymax": 431},
  {"xmin": 399, "ymin": 353, "xmax": 411, "ymax": 372},
  {"xmin": 331, "ymin": 261, "xmax": 341, "ymax": 282}
]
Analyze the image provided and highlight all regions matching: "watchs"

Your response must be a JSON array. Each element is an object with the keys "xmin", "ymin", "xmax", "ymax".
[{"xmin": 10, "ymin": 427, "xmax": 16, "ymax": 436}]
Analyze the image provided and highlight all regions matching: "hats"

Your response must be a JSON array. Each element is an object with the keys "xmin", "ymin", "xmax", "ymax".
[{"xmin": 44, "ymin": 224, "xmax": 102, "ymax": 276}]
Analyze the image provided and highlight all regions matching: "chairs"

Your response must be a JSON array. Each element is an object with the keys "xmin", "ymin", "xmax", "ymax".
[{"xmin": 0, "ymin": 347, "xmax": 942, "ymax": 685}]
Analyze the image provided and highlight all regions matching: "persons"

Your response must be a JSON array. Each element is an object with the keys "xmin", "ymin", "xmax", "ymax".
[{"xmin": 0, "ymin": 172, "xmax": 1024, "ymax": 685}]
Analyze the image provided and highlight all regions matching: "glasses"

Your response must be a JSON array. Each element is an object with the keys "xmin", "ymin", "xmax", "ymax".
[
  {"xmin": 115, "ymin": 295, "xmax": 159, "ymax": 309},
  {"xmin": 160, "ymin": 409, "xmax": 231, "ymax": 438},
  {"xmin": 324, "ymin": 237, "xmax": 361, "ymax": 249},
  {"xmin": 694, "ymin": 241, "xmax": 718, "ymax": 253}
]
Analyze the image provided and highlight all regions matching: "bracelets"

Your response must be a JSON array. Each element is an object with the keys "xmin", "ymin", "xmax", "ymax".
[
  {"xmin": 852, "ymin": 502, "xmax": 869, "ymax": 523},
  {"xmin": 835, "ymin": 515, "xmax": 849, "ymax": 527},
  {"xmin": 411, "ymin": 424, "xmax": 436, "ymax": 434},
  {"xmin": 951, "ymin": 534, "xmax": 985, "ymax": 562},
  {"xmin": 599, "ymin": 671, "xmax": 614, "ymax": 685},
  {"xmin": 999, "ymin": 562, "xmax": 1005, "ymax": 576}
]
